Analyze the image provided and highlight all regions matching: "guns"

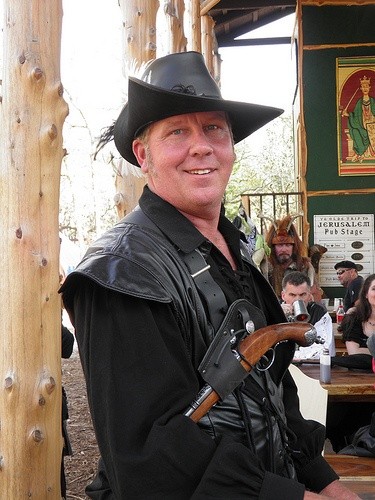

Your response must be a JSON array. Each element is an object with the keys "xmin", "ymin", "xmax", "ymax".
[{"xmin": 185, "ymin": 322, "xmax": 324, "ymax": 423}]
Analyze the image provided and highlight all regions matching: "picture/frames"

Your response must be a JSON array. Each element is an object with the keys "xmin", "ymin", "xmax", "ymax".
[{"xmin": 336, "ymin": 56, "xmax": 375, "ymax": 177}]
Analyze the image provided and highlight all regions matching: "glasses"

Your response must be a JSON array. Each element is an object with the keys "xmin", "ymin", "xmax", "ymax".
[{"xmin": 337, "ymin": 269, "xmax": 351, "ymax": 275}]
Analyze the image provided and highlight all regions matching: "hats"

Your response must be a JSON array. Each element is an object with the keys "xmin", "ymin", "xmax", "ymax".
[
  {"xmin": 114, "ymin": 52, "xmax": 284, "ymax": 168},
  {"xmin": 335, "ymin": 261, "xmax": 355, "ymax": 269}
]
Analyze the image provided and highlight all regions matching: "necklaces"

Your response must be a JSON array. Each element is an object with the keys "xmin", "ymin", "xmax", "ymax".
[{"xmin": 367, "ymin": 319, "xmax": 375, "ymax": 326}]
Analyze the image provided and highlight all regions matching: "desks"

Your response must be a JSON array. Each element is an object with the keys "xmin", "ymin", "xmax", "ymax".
[{"xmin": 287, "ymin": 361, "xmax": 375, "ymax": 457}]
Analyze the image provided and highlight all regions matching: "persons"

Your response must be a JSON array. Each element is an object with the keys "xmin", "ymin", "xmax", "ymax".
[
  {"xmin": 255, "ymin": 214, "xmax": 375, "ymax": 455},
  {"xmin": 58, "ymin": 51, "xmax": 363, "ymax": 500}
]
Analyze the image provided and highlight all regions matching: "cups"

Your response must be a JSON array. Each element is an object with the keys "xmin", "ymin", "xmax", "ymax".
[
  {"xmin": 289, "ymin": 299, "xmax": 311, "ymax": 323},
  {"xmin": 334, "ymin": 297, "xmax": 343, "ymax": 311},
  {"xmin": 321, "ymin": 298, "xmax": 329, "ymax": 310}
]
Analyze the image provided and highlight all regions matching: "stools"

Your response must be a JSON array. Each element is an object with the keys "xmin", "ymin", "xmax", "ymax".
[{"xmin": 320, "ymin": 458, "xmax": 375, "ymax": 481}]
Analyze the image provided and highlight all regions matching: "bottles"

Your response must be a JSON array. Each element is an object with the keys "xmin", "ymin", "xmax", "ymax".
[
  {"xmin": 337, "ymin": 305, "xmax": 346, "ymax": 326},
  {"xmin": 320, "ymin": 349, "xmax": 331, "ymax": 384}
]
